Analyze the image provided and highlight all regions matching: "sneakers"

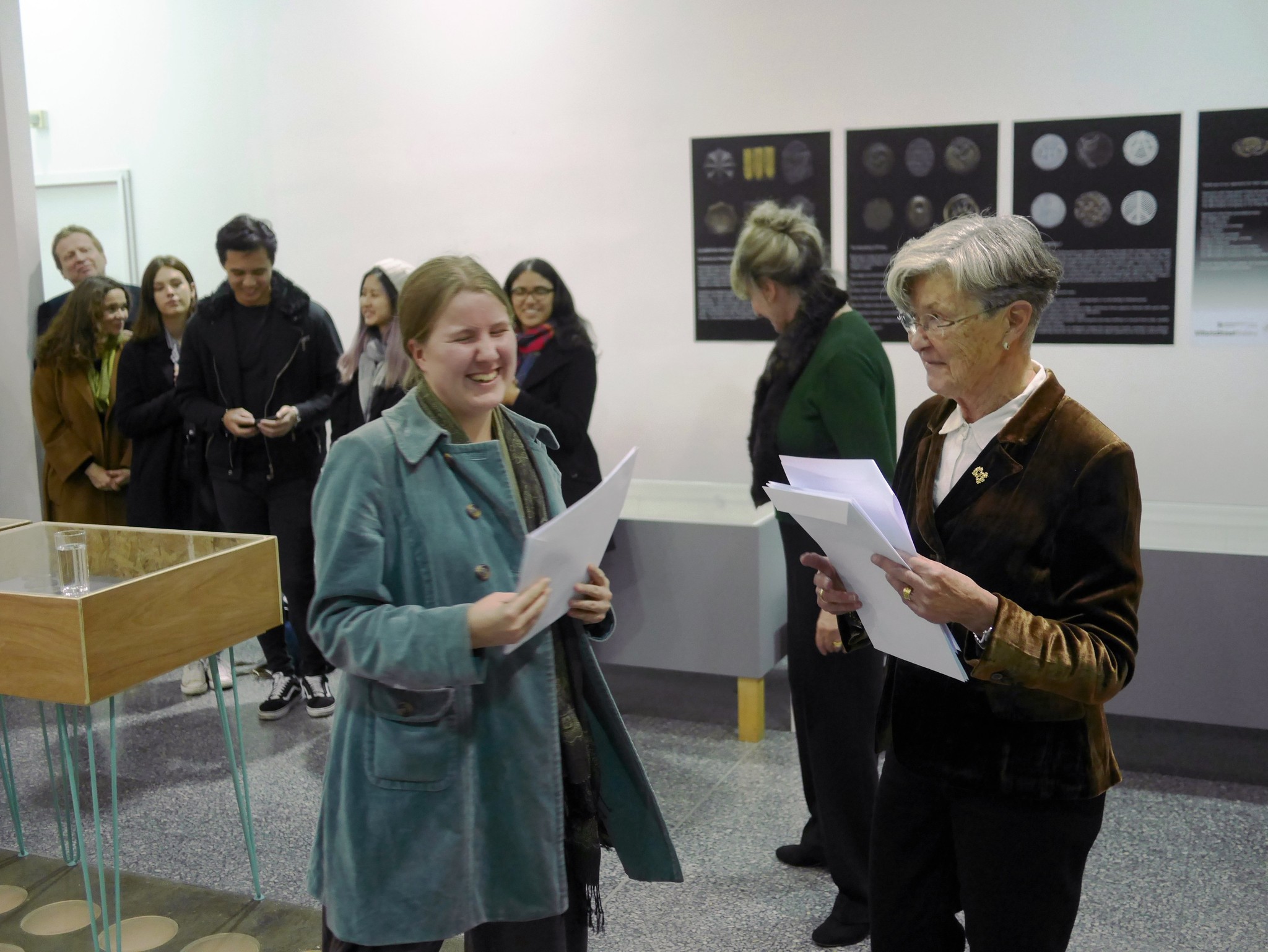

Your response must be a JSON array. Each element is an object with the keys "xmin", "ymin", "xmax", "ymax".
[
  {"xmin": 181, "ymin": 660, "xmax": 207, "ymax": 695},
  {"xmin": 256, "ymin": 671, "xmax": 302, "ymax": 721},
  {"xmin": 299, "ymin": 675, "xmax": 336, "ymax": 717},
  {"xmin": 206, "ymin": 651, "xmax": 233, "ymax": 689}
]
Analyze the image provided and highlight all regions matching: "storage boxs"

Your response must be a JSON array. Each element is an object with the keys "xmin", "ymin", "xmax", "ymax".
[{"xmin": 0, "ymin": 521, "xmax": 286, "ymax": 706}]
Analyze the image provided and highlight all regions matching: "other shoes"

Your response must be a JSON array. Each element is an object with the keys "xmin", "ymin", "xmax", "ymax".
[
  {"xmin": 811, "ymin": 877, "xmax": 883, "ymax": 946},
  {"xmin": 777, "ymin": 843, "xmax": 820, "ymax": 869}
]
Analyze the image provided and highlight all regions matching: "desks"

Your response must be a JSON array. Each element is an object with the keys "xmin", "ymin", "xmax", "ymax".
[
  {"xmin": 599, "ymin": 481, "xmax": 790, "ymax": 742},
  {"xmin": 1097, "ymin": 505, "xmax": 1268, "ymax": 732}
]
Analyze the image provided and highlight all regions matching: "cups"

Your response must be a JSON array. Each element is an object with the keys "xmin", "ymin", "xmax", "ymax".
[{"xmin": 54, "ymin": 529, "xmax": 90, "ymax": 597}]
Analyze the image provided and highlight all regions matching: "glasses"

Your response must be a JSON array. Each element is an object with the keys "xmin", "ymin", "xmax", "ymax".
[
  {"xmin": 515, "ymin": 286, "xmax": 554, "ymax": 302},
  {"xmin": 896, "ymin": 301, "xmax": 999, "ymax": 340}
]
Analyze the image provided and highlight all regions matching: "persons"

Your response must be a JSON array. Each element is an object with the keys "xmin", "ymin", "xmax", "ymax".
[
  {"xmin": 306, "ymin": 254, "xmax": 691, "ymax": 951},
  {"xmin": 30, "ymin": 275, "xmax": 136, "ymax": 528},
  {"xmin": 116, "ymin": 253, "xmax": 236, "ymax": 698},
  {"xmin": 328, "ymin": 261, "xmax": 412, "ymax": 445},
  {"xmin": 37, "ymin": 226, "xmax": 142, "ymax": 338},
  {"xmin": 730, "ymin": 199, "xmax": 897, "ymax": 947},
  {"xmin": 799, "ymin": 214, "xmax": 1143, "ymax": 951},
  {"xmin": 174, "ymin": 212, "xmax": 348, "ymax": 720},
  {"xmin": 502, "ymin": 257, "xmax": 620, "ymax": 555}
]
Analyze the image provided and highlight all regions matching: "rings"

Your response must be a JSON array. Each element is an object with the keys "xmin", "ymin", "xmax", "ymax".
[
  {"xmin": 819, "ymin": 588, "xmax": 827, "ymax": 604},
  {"xmin": 902, "ymin": 586, "xmax": 913, "ymax": 601},
  {"xmin": 833, "ymin": 641, "xmax": 842, "ymax": 647}
]
